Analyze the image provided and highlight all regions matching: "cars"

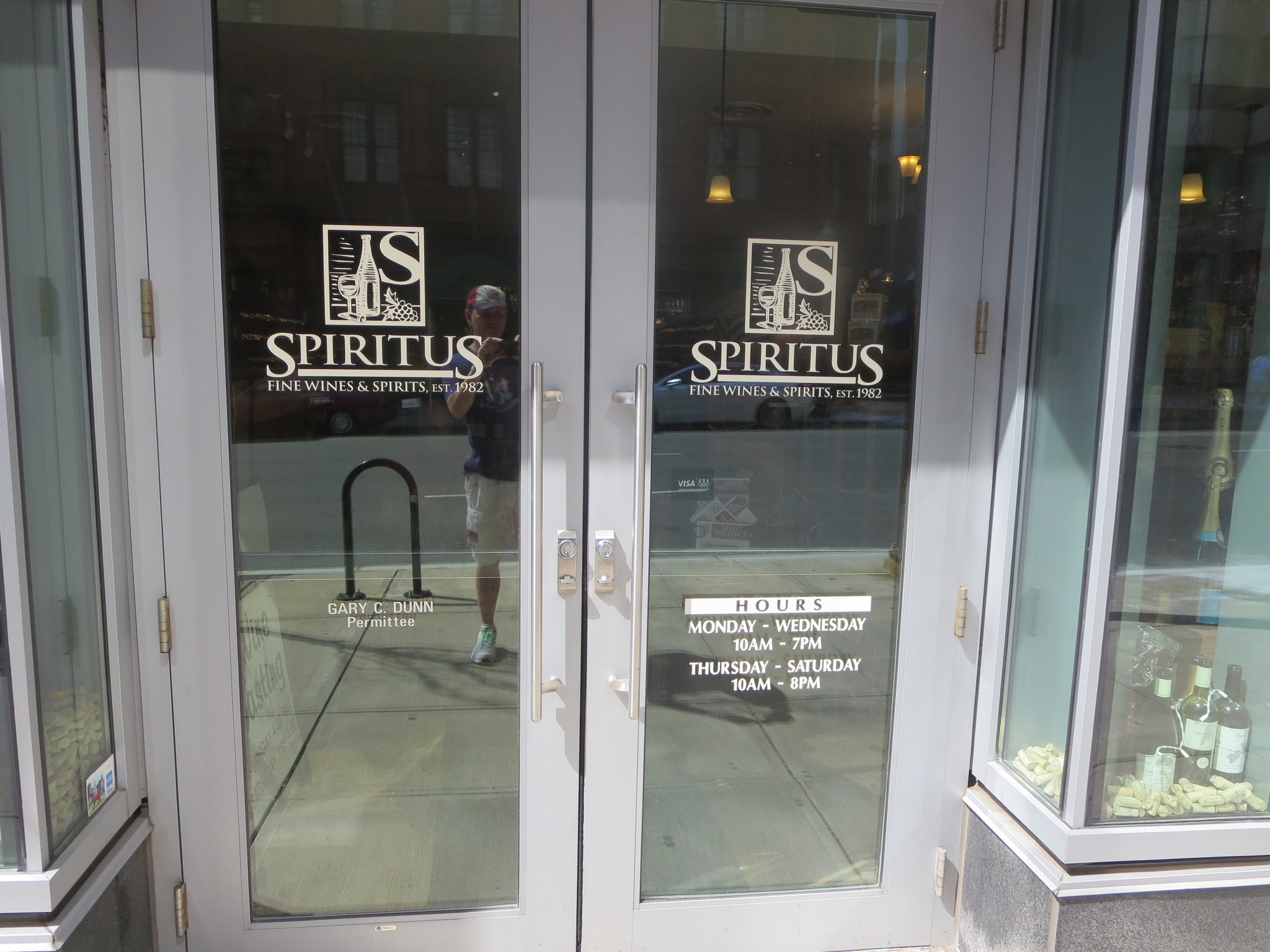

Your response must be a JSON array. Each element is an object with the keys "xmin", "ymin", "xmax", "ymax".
[
  {"xmin": 229, "ymin": 355, "xmax": 379, "ymax": 436},
  {"xmin": 649, "ymin": 363, "xmax": 816, "ymax": 432}
]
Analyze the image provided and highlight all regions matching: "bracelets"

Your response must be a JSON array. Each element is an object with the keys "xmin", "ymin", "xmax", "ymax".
[{"xmin": 471, "ymin": 365, "xmax": 482, "ymax": 375}]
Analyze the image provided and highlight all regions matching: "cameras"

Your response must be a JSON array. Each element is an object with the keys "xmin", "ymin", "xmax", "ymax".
[{"xmin": 500, "ymin": 341, "xmax": 519, "ymax": 354}]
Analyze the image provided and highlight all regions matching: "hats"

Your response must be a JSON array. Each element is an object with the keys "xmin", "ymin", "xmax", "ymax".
[{"xmin": 467, "ymin": 285, "xmax": 509, "ymax": 311}]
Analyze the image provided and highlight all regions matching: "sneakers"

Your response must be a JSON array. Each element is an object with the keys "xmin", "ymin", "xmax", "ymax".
[{"xmin": 470, "ymin": 623, "xmax": 500, "ymax": 664}]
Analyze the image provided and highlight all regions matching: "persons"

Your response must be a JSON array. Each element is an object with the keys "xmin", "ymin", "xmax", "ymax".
[{"xmin": 440, "ymin": 283, "xmax": 521, "ymax": 667}]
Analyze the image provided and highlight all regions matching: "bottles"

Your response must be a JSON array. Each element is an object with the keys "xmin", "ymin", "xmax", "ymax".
[
  {"xmin": 1133, "ymin": 654, "xmax": 1249, "ymax": 796},
  {"xmin": 1170, "ymin": 383, "xmax": 1236, "ymax": 624}
]
